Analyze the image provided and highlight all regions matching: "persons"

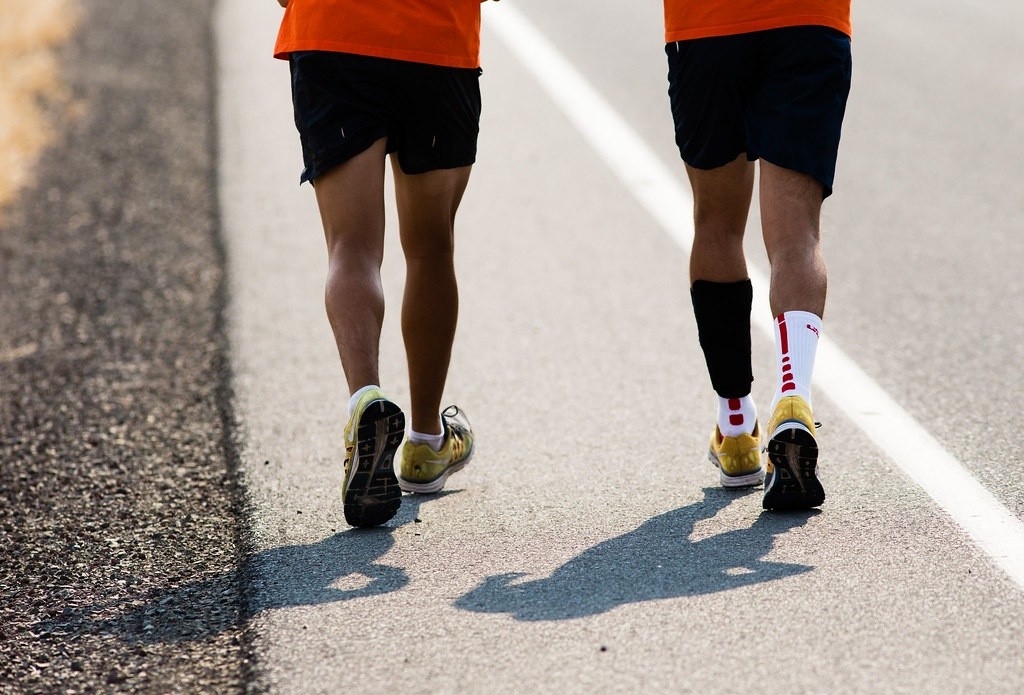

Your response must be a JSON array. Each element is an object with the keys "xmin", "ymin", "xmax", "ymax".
[
  {"xmin": 663, "ymin": 0, "xmax": 853, "ymax": 510},
  {"xmin": 274, "ymin": 0, "xmax": 500, "ymax": 528}
]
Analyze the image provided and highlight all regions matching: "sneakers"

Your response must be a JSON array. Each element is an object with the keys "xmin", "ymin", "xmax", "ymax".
[
  {"xmin": 398, "ymin": 405, "xmax": 474, "ymax": 494},
  {"xmin": 761, "ymin": 396, "xmax": 825, "ymax": 512},
  {"xmin": 342, "ymin": 389, "xmax": 406, "ymax": 528},
  {"xmin": 708, "ymin": 419, "xmax": 765, "ymax": 487}
]
